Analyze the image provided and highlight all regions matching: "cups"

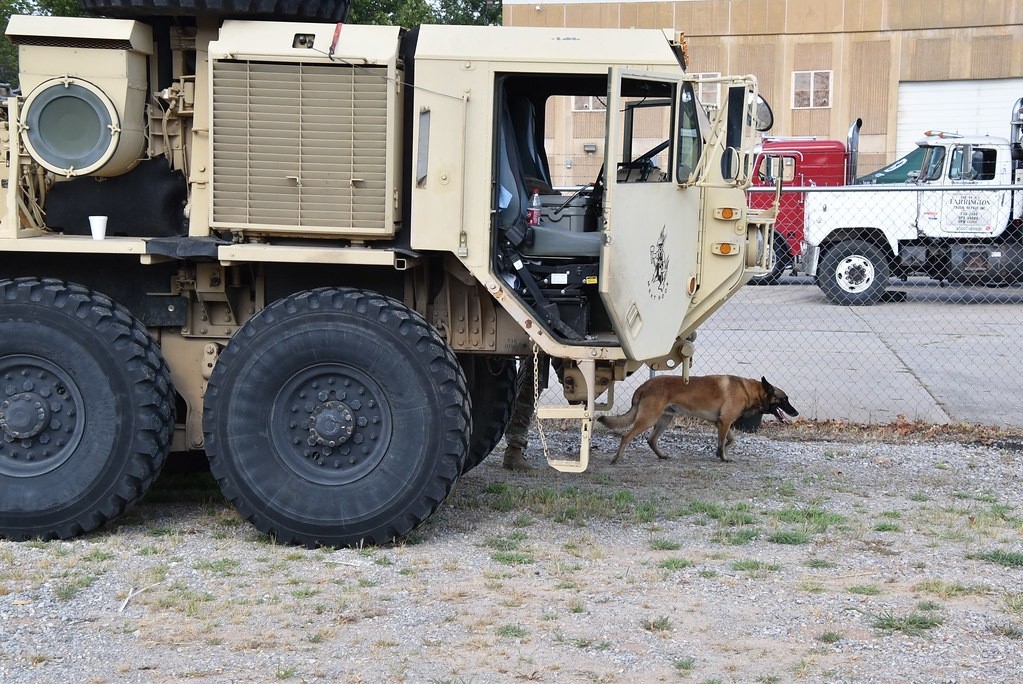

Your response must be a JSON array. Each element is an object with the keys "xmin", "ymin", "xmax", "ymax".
[{"xmin": 88, "ymin": 215, "xmax": 108, "ymax": 240}]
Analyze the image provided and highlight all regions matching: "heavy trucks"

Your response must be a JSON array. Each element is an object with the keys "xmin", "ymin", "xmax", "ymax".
[
  {"xmin": 745, "ymin": 97, "xmax": 1023, "ymax": 306},
  {"xmin": 0, "ymin": 1, "xmax": 781, "ymax": 551}
]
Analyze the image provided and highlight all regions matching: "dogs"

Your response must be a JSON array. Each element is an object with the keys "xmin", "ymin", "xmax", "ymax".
[{"xmin": 596, "ymin": 374, "xmax": 800, "ymax": 466}]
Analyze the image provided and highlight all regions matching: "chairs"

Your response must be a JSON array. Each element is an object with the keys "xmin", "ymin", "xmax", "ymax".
[
  {"xmin": 514, "ymin": 96, "xmax": 562, "ymax": 201},
  {"xmin": 501, "ymin": 117, "xmax": 601, "ymax": 259}
]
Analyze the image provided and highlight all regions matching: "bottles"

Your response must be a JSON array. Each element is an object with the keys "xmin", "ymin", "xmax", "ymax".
[{"xmin": 527, "ymin": 189, "xmax": 541, "ymax": 227}]
